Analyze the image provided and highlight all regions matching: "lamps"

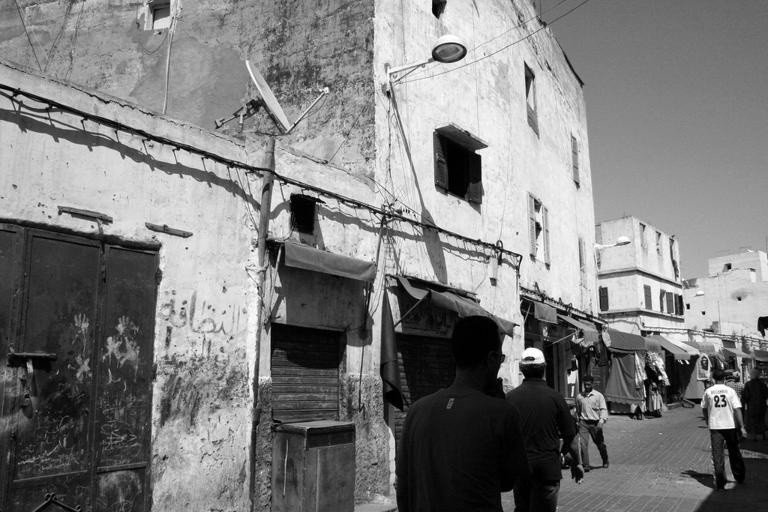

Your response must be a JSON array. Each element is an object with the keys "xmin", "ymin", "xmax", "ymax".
[
  {"xmin": 594, "ymin": 236, "xmax": 631, "ymax": 249},
  {"xmin": 386, "ymin": 35, "xmax": 467, "ymax": 95},
  {"xmin": 686, "ymin": 290, "xmax": 705, "ymax": 298}
]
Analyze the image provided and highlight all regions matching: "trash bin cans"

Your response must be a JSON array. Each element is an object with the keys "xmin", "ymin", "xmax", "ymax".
[
  {"xmin": 271, "ymin": 421, "xmax": 356, "ymax": 512},
  {"xmin": 559, "ymin": 398, "xmax": 581, "ymax": 468}
]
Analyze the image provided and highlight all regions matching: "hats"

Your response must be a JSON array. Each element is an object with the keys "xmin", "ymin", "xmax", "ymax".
[{"xmin": 520, "ymin": 348, "xmax": 545, "ymax": 366}]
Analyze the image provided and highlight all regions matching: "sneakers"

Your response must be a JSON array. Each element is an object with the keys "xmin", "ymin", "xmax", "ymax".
[
  {"xmin": 584, "ymin": 465, "xmax": 590, "ymax": 472},
  {"xmin": 603, "ymin": 460, "xmax": 610, "ymax": 468}
]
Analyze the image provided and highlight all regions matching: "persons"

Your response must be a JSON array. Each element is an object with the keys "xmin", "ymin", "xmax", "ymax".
[
  {"xmin": 393, "ymin": 314, "xmax": 535, "ymax": 512},
  {"xmin": 575, "ymin": 375, "xmax": 610, "ymax": 472},
  {"xmin": 700, "ymin": 368, "xmax": 748, "ymax": 492},
  {"xmin": 725, "ymin": 372, "xmax": 741, "ymax": 398},
  {"xmin": 504, "ymin": 346, "xmax": 585, "ymax": 512},
  {"xmin": 742, "ymin": 368, "xmax": 768, "ymax": 441},
  {"xmin": 734, "ymin": 376, "xmax": 744, "ymax": 388}
]
